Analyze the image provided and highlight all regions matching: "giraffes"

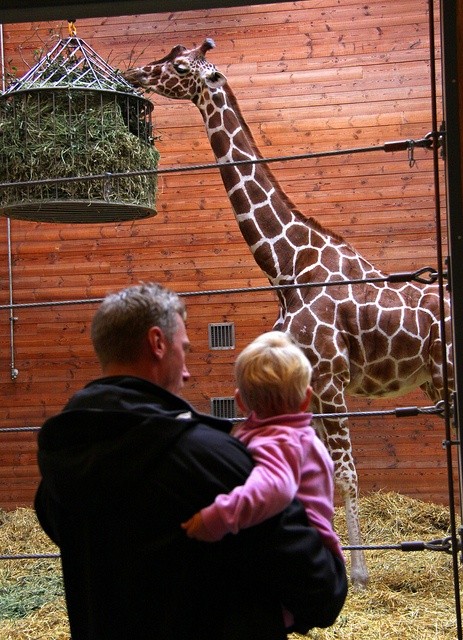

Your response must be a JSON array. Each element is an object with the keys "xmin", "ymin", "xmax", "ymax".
[{"xmin": 121, "ymin": 38, "xmax": 458, "ymax": 588}]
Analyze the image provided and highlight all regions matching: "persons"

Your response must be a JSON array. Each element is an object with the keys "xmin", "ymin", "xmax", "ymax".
[
  {"xmin": 33, "ymin": 281, "xmax": 347, "ymax": 640},
  {"xmin": 179, "ymin": 330, "xmax": 346, "ymax": 628}
]
